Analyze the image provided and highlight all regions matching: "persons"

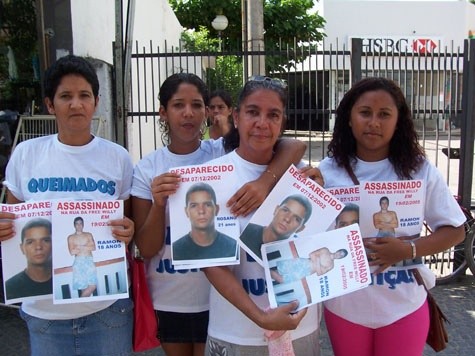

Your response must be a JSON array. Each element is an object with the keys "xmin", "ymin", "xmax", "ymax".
[
  {"xmin": 173, "ymin": 183, "xmax": 236, "ymax": 260},
  {"xmin": 5, "ymin": 217, "xmax": 53, "ymax": 300},
  {"xmin": 373, "ymin": 196, "xmax": 398, "ymax": 266},
  {"xmin": 270, "ymin": 247, "xmax": 348, "ymax": 285},
  {"xmin": 67, "ymin": 217, "xmax": 97, "ymax": 297},
  {"xmin": 130, "ymin": 72, "xmax": 306, "ymax": 356},
  {"xmin": 317, "ymin": 76, "xmax": 467, "ymax": 356},
  {"xmin": 192, "ymin": 77, "xmax": 324, "ymax": 356},
  {"xmin": 202, "ymin": 90, "xmax": 233, "ymax": 139},
  {"xmin": 334, "ymin": 202, "xmax": 359, "ymax": 229},
  {"xmin": 0, "ymin": 55, "xmax": 134, "ymax": 356},
  {"xmin": 240, "ymin": 194, "xmax": 312, "ymax": 261}
]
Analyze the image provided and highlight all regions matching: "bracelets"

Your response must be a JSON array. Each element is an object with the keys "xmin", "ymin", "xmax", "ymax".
[
  {"xmin": 410, "ymin": 240, "xmax": 416, "ymax": 259},
  {"xmin": 267, "ymin": 169, "xmax": 279, "ymax": 183}
]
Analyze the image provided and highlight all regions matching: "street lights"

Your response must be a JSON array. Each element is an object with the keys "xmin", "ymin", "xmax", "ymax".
[{"xmin": 211, "ymin": 15, "xmax": 228, "ymax": 53}]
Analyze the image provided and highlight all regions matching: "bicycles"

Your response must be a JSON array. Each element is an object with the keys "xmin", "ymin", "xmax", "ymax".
[{"xmin": 421, "ymin": 194, "xmax": 475, "ymax": 285}]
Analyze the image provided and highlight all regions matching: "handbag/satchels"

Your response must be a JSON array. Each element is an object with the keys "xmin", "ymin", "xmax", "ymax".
[{"xmin": 425, "ymin": 294, "xmax": 451, "ymax": 352}]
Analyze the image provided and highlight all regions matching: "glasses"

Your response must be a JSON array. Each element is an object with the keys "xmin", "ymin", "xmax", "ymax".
[{"xmin": 244, "ymin": 75, "xmax": 288, "ymax": 94}]
